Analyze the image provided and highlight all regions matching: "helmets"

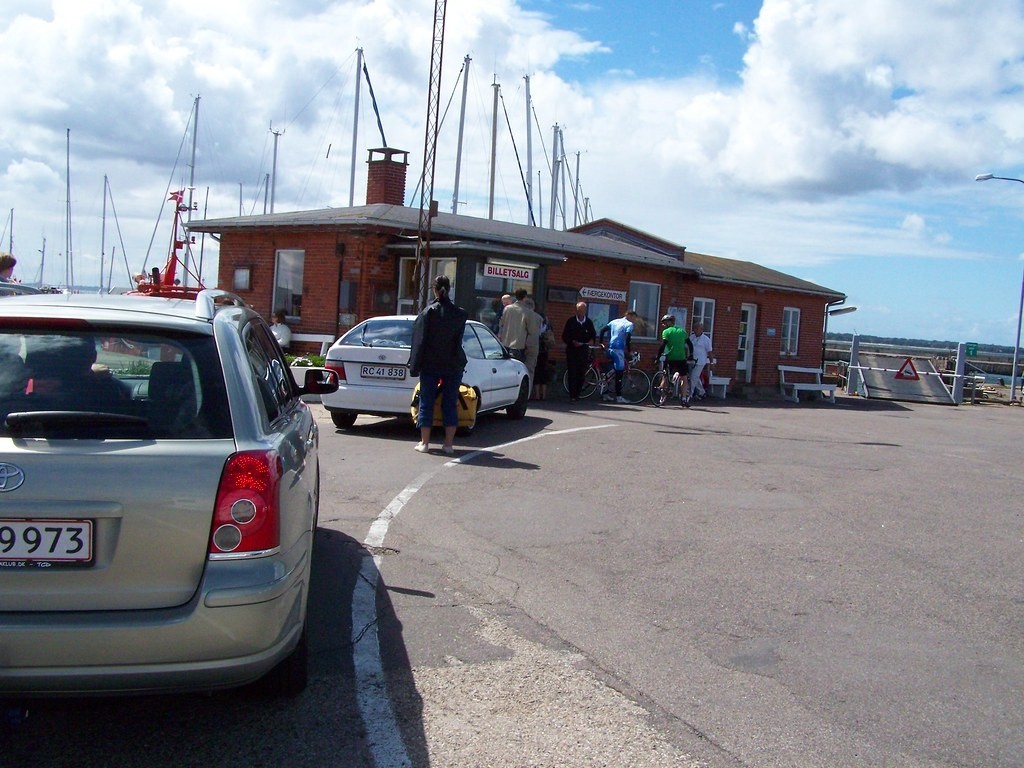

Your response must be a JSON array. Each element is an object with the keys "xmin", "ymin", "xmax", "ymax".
[{"xmin": 661, "ymin": 315, "xmax": 676, "ymax": 322}]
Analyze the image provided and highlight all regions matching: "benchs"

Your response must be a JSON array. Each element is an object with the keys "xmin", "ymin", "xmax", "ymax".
[
  {"xmin": 778, "ymin": 365, "xmax": 838, "ymax": 404},
  {"xmin": 706, "ymin": 358, "xmax": 730, "ymax": 399}
]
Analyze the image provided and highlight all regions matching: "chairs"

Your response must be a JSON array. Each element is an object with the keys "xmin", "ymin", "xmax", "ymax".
[{"xmin": 142, "ymin": 361, "xmax": 193, "ymax": 422}]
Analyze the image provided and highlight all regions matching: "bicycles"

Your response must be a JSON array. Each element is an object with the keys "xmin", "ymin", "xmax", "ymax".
[
  {"xmin": 648, "ymin": 353, "xmax": 700, "ymax": 405},
  {"xmin": 562, "ymin": 344, "xmax": 651, "ymax": 404}
]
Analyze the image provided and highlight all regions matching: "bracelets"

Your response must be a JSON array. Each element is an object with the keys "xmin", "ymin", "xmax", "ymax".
[{"xmin": 711, "ymin": 363, "xmax": 713, "ymax": 364}]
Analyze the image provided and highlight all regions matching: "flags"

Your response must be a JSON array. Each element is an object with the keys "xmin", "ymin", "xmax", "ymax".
[{"xmin": 167, "ymin": 191, "xmax": 184, "ymax": 203}]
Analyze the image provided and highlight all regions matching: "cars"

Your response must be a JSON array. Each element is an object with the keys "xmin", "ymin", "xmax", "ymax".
[{"xmin": 319, "ymin": 314, "xmax": 535, "ymax": 430}]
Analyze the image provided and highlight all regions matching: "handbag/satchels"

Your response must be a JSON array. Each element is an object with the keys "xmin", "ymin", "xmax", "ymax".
[
  {"xmin": 543, "ymin": 330, "xmax": 555, "ymax": 349},
  {"xmin": 410, "ymin": 383, "xmax": 478, "ymax": 430}
]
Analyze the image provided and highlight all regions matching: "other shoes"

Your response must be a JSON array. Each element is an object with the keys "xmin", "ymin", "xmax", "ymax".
[
  {"xmin": 570, "ymin": 397, "xmax": 576, "ymax": 404},
  {"xmin": 694, "ymin": 392, "xmax": 706, "ymax": 401},
  {"xmin": 617, "ymin": 397, "xmax": 631, "ymax": 404},
  {"xmin": 682, "ymin": 395, "xmax": 690, "ymax": 405},
  {"xmin": 575, "ymin": 396, "xmax": 579, "ymax": 401},
  {"xmin": 414, "ymin": 443, "xmax": 428, "ymax": 453},
  {"xmin": 660, "ymin": 396, "xmax": 666, "ymax": 404},
  {"xmin": 602, "ymin": 395, "xmax": 614, "ymax": 400},
  {"xmin": 441, "ymin": 444, "xmax": 453, "ymax": 456}
]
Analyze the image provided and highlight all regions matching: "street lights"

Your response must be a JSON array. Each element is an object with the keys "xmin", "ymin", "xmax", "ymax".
[{"xmin": 974, "ymin": 172, "xmax": 1024, "ymax": 405}]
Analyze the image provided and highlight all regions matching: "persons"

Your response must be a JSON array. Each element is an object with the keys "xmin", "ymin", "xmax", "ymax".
[
  {"xmin": 686, "ymin": 323, "xmax": 714, "ymax": 402},
  {"xmin": 13, "ymin": 278, "xmax": 21, "ymax": 284},
  {"xmin": 561, "ymin": 302, "xmax": 596, "ymax": 405},
  {"xmin": 653, "ymin": 314, "xmax": 694, "ymax": 408},
  {"xmin": 599, "ymin": 311, "xmax": 637, "ymax": 403},
  {"xmin": 0, "ymin": 253, "xmax": 21, "ymax": 296},
  {"xmin": 410, "ymin": 275, "xmax": 468, "ymax": 453},
  {"xmin": 26, "ymin": 362, "xmax": 112, "ymax": 394},
  {"xmin": 269, "ymin": 311, "xmax": 291, "ymax": 356},
  {"xmin": 493, "ymin": 288, "xmax": 552, "ymax": 400}
]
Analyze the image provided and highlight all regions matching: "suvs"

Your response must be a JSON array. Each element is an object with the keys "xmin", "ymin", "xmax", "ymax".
[{"xmin": 0, "ymin": 283, "xmax": 340, "ymax": 696}]
